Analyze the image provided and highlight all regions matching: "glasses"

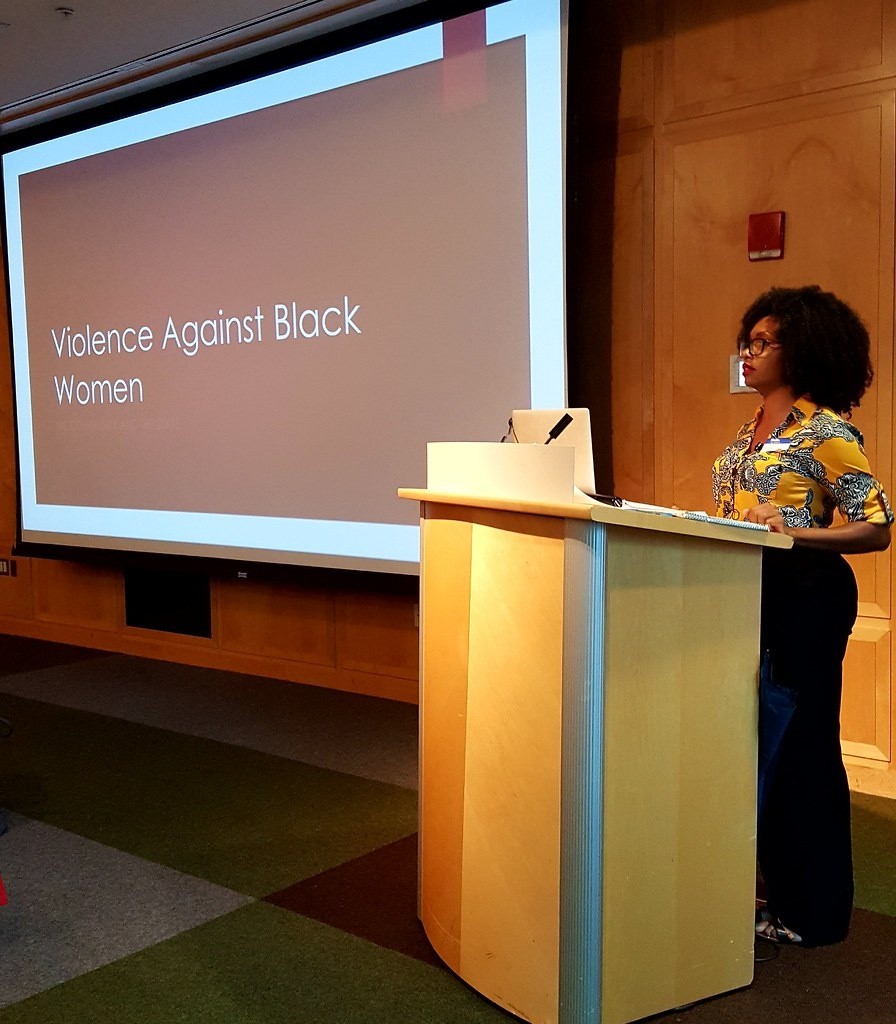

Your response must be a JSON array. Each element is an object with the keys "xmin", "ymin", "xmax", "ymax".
[{"xmin": 737, "ymin": 337, "xmax": 777, "ymax": 356}]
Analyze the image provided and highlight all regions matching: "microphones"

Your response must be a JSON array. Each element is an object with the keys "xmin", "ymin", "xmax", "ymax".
[
  {"xmin": 755, "ymin": 442, "xmax": 764, "ymax": 452},
  {"xmin": 544, "ymin": 414, "xmax": 573, "ymax": 445}
]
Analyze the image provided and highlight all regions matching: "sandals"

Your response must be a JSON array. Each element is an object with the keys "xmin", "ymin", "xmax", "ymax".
[{"xmin": 755, "ymin": 917, "xmax": 809, "ymax": 946}]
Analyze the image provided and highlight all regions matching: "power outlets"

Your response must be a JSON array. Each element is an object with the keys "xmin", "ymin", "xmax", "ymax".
[{"xmin": 0, "ymin": 559, "xmax": 9, "ymax": 575}]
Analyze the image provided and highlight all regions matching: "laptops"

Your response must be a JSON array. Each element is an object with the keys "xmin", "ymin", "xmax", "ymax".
[{"xmin": 514, "ymin": 409, "xmax": 622, "ymax": 506}]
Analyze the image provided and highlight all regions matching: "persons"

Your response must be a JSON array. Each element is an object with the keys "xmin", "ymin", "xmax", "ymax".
[{"xmin": 710, "ymin": 282, "xmax": 895, "ymax": 946}]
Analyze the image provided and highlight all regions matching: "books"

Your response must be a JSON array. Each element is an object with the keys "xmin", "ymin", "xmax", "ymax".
[{"xmin": 622, "ymin": 498, "xmax": 770, "ymax": 533}]
